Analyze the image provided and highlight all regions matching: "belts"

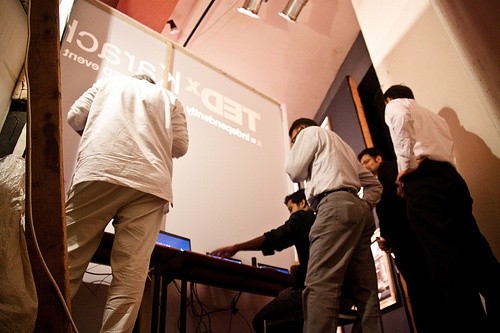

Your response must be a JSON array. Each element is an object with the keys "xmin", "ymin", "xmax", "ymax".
[{"xmin": 313, "ymin": 188, "xmax": 357, "ymax": 210}]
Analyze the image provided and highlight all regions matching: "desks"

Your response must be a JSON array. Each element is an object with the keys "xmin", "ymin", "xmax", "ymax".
[
  {"xmin": 88, "ymin": 231, "xmax": 182, "ymax": 333},
  {"xmin": 159, "ymin": 250, "xmax": 293, "ymax": 333}
]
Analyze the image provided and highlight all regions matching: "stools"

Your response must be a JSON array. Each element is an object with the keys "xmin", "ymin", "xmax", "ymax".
[{"xmin": 266, "ymin": 311, "xmax": 359, "ymax": 333}]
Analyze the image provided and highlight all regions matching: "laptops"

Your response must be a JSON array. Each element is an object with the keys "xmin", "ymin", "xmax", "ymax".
[
  {"xmin": 206, "ymin": 252, "xmax": 242, "ymax": 264},
  {"xmin": 258, "ymin": 263, "xmax": 290, "ymax": 274},
  {"xmin": 156, "ymin": 230, "xmax": 191, "ymax": 252}
]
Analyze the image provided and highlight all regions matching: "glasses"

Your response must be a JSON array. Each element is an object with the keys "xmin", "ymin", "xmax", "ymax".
[
  {"xmin": 361, "ymin": 157, "xmax": 372, "ymax": 166},
  {"xmin": 291, "ymin": 128, "xmax": 303, "ymax": 143}
]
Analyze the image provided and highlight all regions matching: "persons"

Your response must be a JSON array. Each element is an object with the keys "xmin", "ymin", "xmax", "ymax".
[
  {"xmin": 64, "ymin": 75, "xmax": 188, "ymax": 333},
  {"xmin": 358, "ymin": 86, "xmax": 500, "ymax": 333},
  {"xmin": 209, "ymin": 117, "xmax": 383, "ymax": 333}
]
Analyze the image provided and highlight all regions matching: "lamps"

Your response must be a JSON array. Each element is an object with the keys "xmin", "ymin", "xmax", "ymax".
[
  {"xmin": 278, "ymin": 0, "xmax": 310, "ymax": 24},
  {"xmin": 237, "ymin": 0, "xmax": 269, "ymax": 19},
  {"xmin": 166, "ymin": 19, "xmax": 182, "ymax": 34}
]
